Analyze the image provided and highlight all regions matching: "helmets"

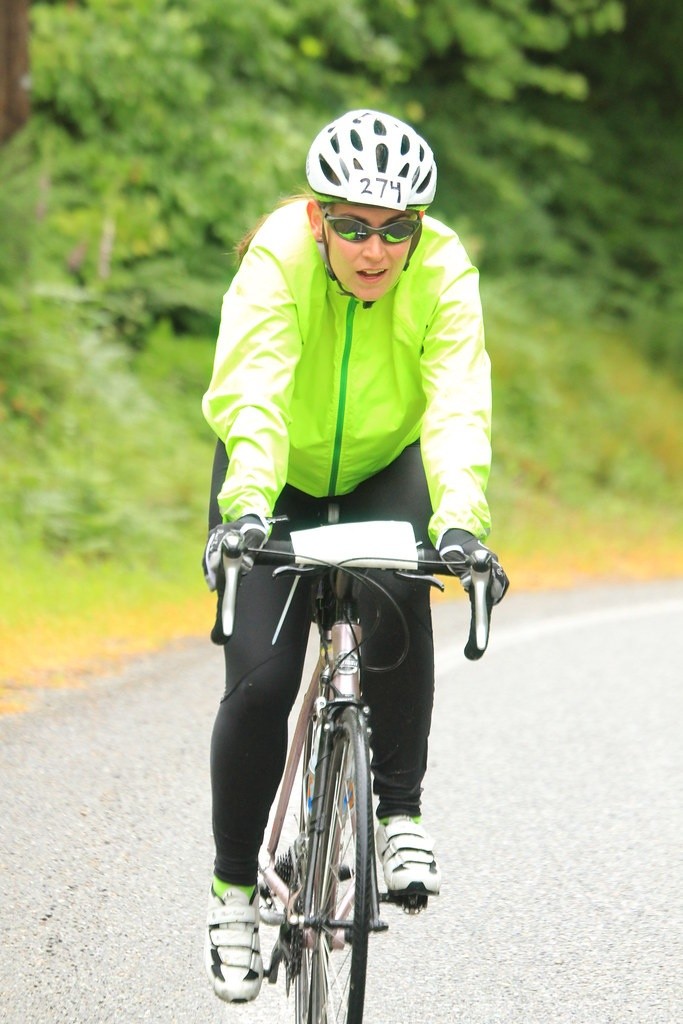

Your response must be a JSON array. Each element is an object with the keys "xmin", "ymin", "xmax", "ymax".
[{"xmin": 306, "ymin": 109, "xmax": 437, "ymax": 211}]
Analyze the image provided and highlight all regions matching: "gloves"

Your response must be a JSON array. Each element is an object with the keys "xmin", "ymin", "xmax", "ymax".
[
  {"xmin": 203, "ymin": 514, "xmax": 267, "ymax": 593},
  {"xmin": 439, "ymin": 528, "xmax": 509, "ymax": 606}
]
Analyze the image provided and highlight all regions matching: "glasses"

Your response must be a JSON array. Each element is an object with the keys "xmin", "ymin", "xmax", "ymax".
[{"xmin": 321, "ymin": 203, "xmax": 422, "ymax": 244}]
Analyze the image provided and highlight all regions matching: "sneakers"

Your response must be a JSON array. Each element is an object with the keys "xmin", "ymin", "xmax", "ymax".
[
  {"xmin": 376, "ymin": 815, "xmax": 442, "ymax": 896},
  {"xmin": 204, "ymin": 882, "xmax": 264, "ymax": 1005}
]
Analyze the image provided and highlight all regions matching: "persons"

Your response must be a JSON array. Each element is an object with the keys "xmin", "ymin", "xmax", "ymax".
[{"xmin": 201, "ymin": 108, "xmax": 511, "ymax": 1006}]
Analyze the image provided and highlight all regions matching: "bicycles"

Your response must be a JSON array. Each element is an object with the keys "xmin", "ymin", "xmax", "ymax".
[{"xmin": 210, "ymin": 532, "xmax": 494, "ymax": 1024}]
